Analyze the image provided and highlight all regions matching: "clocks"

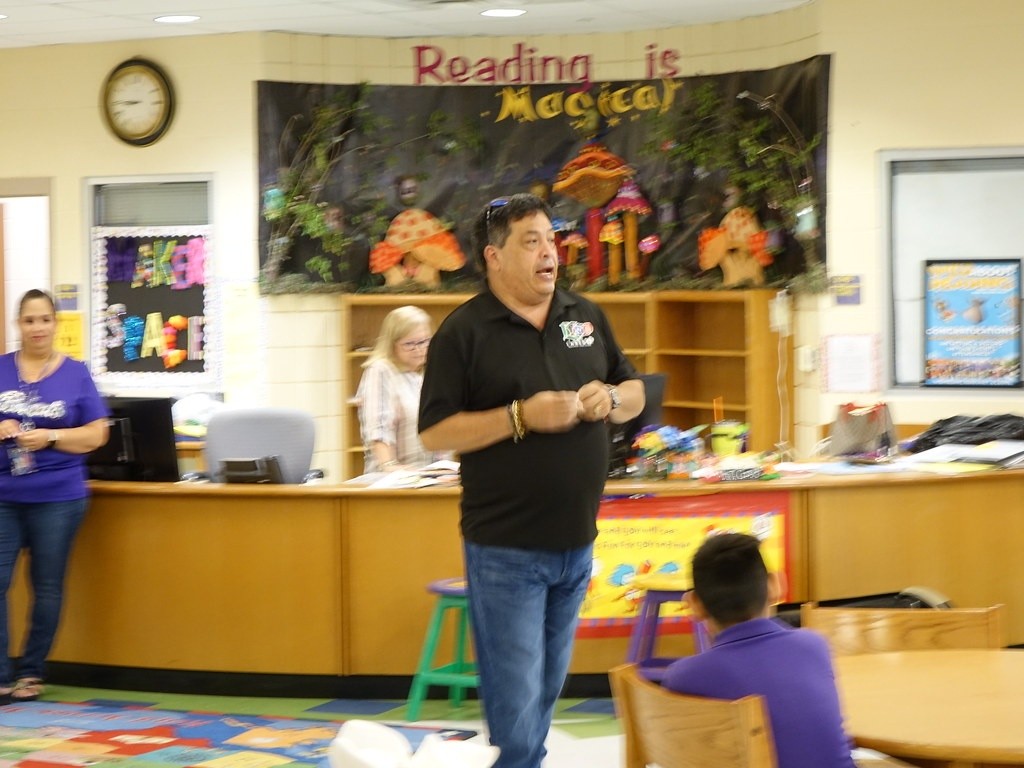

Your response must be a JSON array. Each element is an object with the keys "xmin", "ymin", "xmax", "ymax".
[{"xmin": 102, "ymin": 59, "xmax": 177, "ymax": 147}]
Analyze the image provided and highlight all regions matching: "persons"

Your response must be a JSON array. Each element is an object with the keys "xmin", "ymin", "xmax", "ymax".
[
  {"xmin": 358, "ymin": 306, "xmax": 455, "ymax": 475},
  {"xmin": 418, "ymin": 192, "xmax": 647, "ymax": 768},
  {"xmin": 661, "ymin": 534, "xmax": 858, "ymax": 768},
  {"xmin": 0, "ymin": 289, "xmax": 111, "ymax": 706}
]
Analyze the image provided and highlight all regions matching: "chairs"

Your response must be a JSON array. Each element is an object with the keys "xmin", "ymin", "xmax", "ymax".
[
  {"xmin": 607, "ymin": 663, "xmax": 778, "ymax": 768},
  {"xmin": 799, "ymin": 600, "xmax": 1005, "ymax": 660}
]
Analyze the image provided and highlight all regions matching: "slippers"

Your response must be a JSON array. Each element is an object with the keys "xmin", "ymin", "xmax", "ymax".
[
  {"xmin": 12, "ymin": 679, "xmax": 43, "ymax": 701},
  {"xmin": 0, "ymin": 692, "xmax": 12, "ymax": 705}
]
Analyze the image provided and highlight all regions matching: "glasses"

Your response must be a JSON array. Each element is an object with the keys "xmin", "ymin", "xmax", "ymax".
[
  {"xmin": 487, "ymin": 196, "xmax": 511, "ymax": 245},
  {"xmin": 393, "ymin": 337, "xmax": 431, "ymax": 352}
]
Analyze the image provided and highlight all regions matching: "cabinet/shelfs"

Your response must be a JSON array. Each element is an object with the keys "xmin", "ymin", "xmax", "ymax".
[{"xmin": 344, "ymin": 289, "xmax": 796, "ymax": 481}]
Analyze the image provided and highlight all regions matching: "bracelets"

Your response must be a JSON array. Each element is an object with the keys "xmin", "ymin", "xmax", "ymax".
[{"xmin": 505, "ymin": 398, "xmax": 526, "ymax": 444}]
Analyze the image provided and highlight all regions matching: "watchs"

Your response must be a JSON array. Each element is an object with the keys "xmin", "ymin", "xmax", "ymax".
[
  {"xmin": 46, "ymin": 430, "xmax": 57, "ymax": 451},
  {"xmin": 606, "ymin": 383, "xmax": 621, "ymax": 409}
]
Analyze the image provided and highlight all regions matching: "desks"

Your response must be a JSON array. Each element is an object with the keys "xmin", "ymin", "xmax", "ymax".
[
  {"xmin": 834, "ymin": 650, "xmax": 1024, "ymax": 768},
  {"xmin": 6, "ymin": 441, "xmax": 1024, "ymax": 675}
]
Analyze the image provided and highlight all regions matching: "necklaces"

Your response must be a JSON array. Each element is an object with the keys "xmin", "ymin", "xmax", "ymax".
[{"xmin": 14, "ymin": 352, "xmax": 55, "ymax": 431}]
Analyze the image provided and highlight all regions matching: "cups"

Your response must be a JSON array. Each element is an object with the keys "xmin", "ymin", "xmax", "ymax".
[{"xmin": 710, "ymin": 422, "xmax": 744, "ymax": 457}]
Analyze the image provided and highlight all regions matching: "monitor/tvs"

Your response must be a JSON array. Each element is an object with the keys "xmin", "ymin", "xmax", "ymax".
[
  {"xmin": 607, "ymin": 374, "xmax": 665, "ymax": 470},
  {"xmin": 86, "ymin": 395, "xmax": 179, "ymax": 481}
]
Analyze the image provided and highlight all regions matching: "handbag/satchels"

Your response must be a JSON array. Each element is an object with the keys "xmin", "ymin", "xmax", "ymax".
[{"xmin": 832, "ymin": 396, "xmax": 898, "ymax": 463}]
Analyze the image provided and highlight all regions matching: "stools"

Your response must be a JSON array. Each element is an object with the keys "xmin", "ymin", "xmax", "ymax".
[
  {"xmin": 404, "ymin": 577, "xmax": 480, "ymax": 723},
  {"xmin": 627, "ymin": 574, "xmax": 713, "ymax": 681}
]
